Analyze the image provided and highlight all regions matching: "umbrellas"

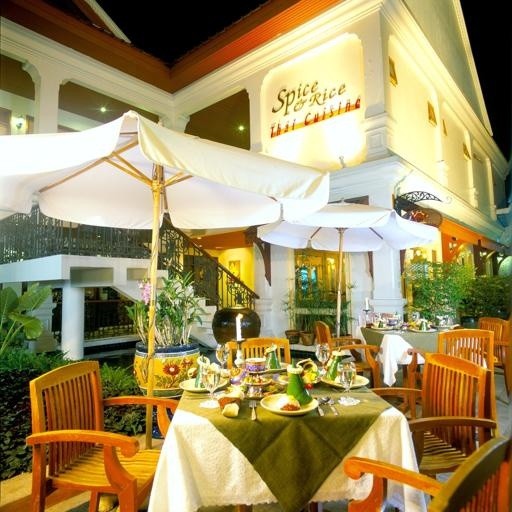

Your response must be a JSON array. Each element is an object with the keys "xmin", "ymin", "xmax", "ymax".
[
  {"xmin": 1, "ymin": 110, "xmax": 329, "ymax": 449},
  {"xmin": 257, "ymin": 198, "xmax": 438, "ymax": 339}
]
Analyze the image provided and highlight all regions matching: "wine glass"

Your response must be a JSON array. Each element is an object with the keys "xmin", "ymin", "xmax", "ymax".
[{"xmin": 199, "ymin": 342, "xmax": 360, "ymax": 408}]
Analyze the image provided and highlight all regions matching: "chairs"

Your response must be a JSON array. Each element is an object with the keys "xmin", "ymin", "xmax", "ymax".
[
  {"xmin": 224, "ymin": 337, "xmax": 291, "ymax": 369},
  {"xmin": 478, "ymin": 317, "xmax": 512, "ymax": 398},
  {"xmin": 370, "ymin": 352, "xmax": 498, "ymax": 500},
  {"xmin": 316, "ymin": 320, "xmax": 381, "ymax": 388},
  {"xmin": 344, "ymin": 438, "xmax": 512, "ymax": 512},
  {"xmin": 407, "ymin": 329, "xmax": 499, "ymax": 437},
  {"xmin": 25, "ymin": 361, "xmax": 179, "ymax": 512}
]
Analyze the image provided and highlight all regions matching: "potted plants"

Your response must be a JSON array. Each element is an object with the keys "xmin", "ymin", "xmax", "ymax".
[
  {"xmin": 281, "ymin": 264, "xmax": 358, "ymax": 347},
  {"xmin": 459, "ymin": 273, "xmax": 512, "ymax": 329},
  {"xmin": 405, "ymin": 262, "xmax": 475, "ymax": 327},
  {"xmin": 125, "ymin": 265, "xmax": 211, "ymax": 397}
]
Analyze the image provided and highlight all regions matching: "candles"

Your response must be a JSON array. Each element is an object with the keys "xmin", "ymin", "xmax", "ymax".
[
  {"xmin": 236, "ymin": 313, "xmax": 243, "ymax": 341},
  {"xmin": 365, "ymin": 298, "xmax": 368, "ymax": 309}
]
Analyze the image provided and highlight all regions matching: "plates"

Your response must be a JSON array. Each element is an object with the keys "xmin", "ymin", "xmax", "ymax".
[
  {"xmin": 179, "ymin": 362, "xmax": 369, "ymax": 416},
  {"xmin": 371, "ymin": 320, "xmax": 460, "ymax": 332}
]
